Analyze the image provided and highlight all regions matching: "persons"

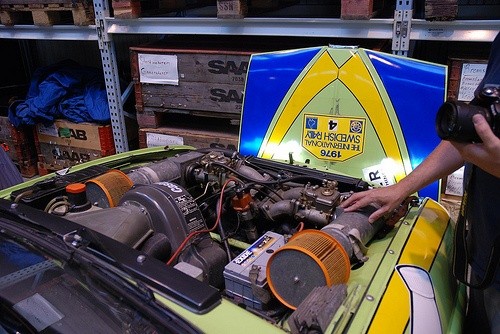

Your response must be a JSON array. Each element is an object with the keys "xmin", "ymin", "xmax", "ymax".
[{"xmin": 341, "ymin": 33, "xmax": 500, "ymax": 334}]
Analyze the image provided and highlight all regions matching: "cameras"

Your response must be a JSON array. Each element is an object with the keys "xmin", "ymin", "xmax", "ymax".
[{"xmin": 434, "ymin": 85, "xmax": 500, "ymax": 143}]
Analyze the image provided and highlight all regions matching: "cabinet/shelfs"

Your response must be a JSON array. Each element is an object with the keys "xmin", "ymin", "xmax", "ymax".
[{"xmin": 0, "ymin": 0, "xmax": 500, "ymax": 219}]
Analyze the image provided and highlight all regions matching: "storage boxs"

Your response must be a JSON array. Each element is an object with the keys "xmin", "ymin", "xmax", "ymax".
[
  {"xmin": 129, "ymin": 46, "xmax": 255, "ymax": 149},
  {"xmin": 0, "ymin": 116, "xmax": 116, "ymax": 178}
]
